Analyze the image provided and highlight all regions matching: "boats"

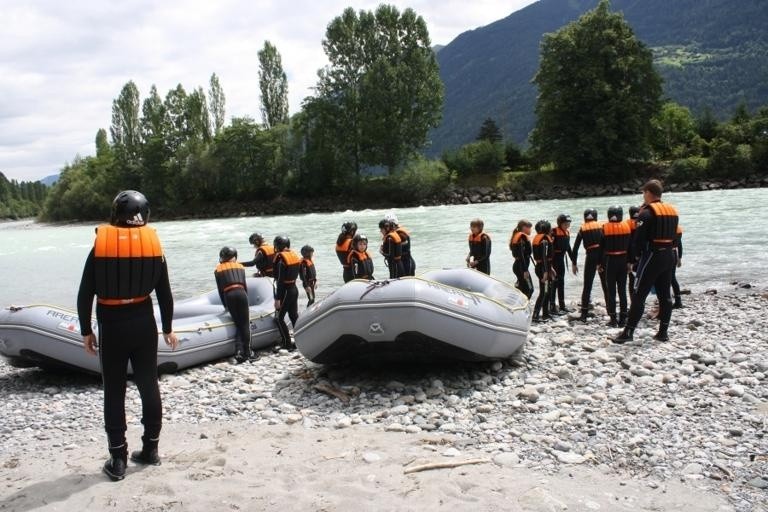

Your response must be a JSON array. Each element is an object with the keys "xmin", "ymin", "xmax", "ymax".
[
  {"xmin": 293, "ymin": 267, "xmax": 532, "ymax": 364},
  {"xmin": 0, "ymin": 276, "xmax": 291, "ymax": 375}
]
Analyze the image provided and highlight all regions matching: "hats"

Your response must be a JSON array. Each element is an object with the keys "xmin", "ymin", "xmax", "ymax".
[{"xmin": 384, "ymin": 213, "xmax": 399, "ymax": 228}]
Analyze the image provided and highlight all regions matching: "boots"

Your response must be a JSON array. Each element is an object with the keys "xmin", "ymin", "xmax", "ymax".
[
  {"xmin": 612, "ymin": 318, "xmax": 671, "ymax": 343},
  {"xmin": 103, "ymin": 444, "xmax": 128, "ymax": 481},
  {"xmin": 574, "ymin": 307, "xmax": 588, "ymax": 322},
  {"xmin": 272, "ymin": 342, "xmax": 294, "ymax": 354},
  {"xmin": 606, "ymin": 312, "xmax": 628, "ymax": 328},
  {"xmin": 532, "ymin": 299, "xmax": 570, "ymax": 323},
  {"xmin": 234, "ymin": 341, "xmax": 261, "ymax": 363},
  {"xmin": 132, "ymin": 436, "xmax": 161, "ymax": 466}
]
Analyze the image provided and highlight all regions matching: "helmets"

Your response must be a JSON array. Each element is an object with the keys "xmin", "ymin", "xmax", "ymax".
[
  {"xmin": 557, "ymin": 204, "xmax": 640, "ymax": 229},
  {"xmin": 535, "ymin": 219, "xmax": 551, "ymax": 234},
  {"xmin": 351, "ymin": 234, "xmax": 368, "ymax": 251},
  {"xmin": 273, "ymin": 235, "xmax": 290, "ymax": 253},
  {"xmin": 301, "ymin": 245, "xmax": 314, "ymax": 257},
  {"xmin": 219, "ymin": 246, "xmax": 238, "ymax": 263},
  {"xmin": 379, "ymin": 219, "xmax": 394, "ymax": 234},
  {"xmin": 111, "ymin": 189, "xmax": 151, "ymax": 227},
  {"xmin": 248, "ymin": 232, "xmax": 264, "ymax": 244},
  {"xmin": 341, "ymin": 221, "xmax": 357, "ymax": 235}
]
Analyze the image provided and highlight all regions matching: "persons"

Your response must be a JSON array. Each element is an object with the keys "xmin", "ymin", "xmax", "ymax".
[
  {"xmin": 334, "ymin": 219, "xmax": 358, "ymax": 283},
  {"xmin": 383, "ymin": 212, "xmax": 417, "ymax": 276},
  {"xmin": 509, "ymin": 179, "xmax": 685, "ymax": 346},
  {"xmin": 299, "ymin": 244, "xmax": 318, "ymax": 308},
  {"xmin": 74, "ymin": 189, "xmax": 180, "ymax": 484},
  {"xmin": 238, "ymin": 232, "xmax": 278, "ymax": 276},
  {"xmin": 214, "ymin": 246, "xmax": 260, "ymax": 362},
  {"xmin": 347, "ymin": 234, "xmax": 375, "ymax": 280},
  {"xmin": 377, "ymin": 218, "xmax": 407, "ymax": 278},
  {"xmin": 464, "ymin": 218, "xmax": 492, "ymax": 277},
  {"xmin": 271, "ymin": 234, "xmax": 305, "ymax": 356}
]
